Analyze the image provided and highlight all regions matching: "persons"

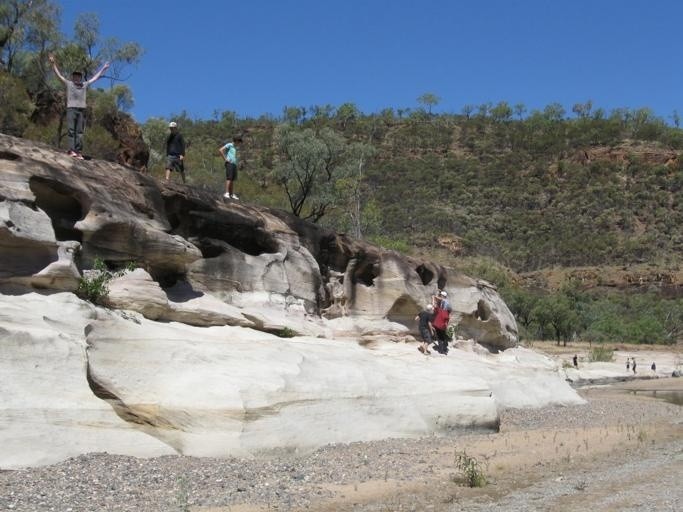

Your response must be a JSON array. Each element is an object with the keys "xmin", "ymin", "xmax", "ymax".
[
  {"xmin": 625, "ymin": 358, "xmax": 630, "ymax": 371},
  {"xmin": 414, "ymin": 292, "xmax": 451, "ymax": 354},
  {"xmin": 165, "ymin": 122, "xmax": 188, "ymax": 185},
  {"xmin": 573, "ymin": 354, "xmax": 578, "ymax": 367},
  {"xmin": 48, "ymin": 56, "xmax": 109, "ymax": 160},
  {"xmin": 632, "ymin": 357, "xmax": 637, "ymax": 374},
  {"xmin": 651, "ymin": 362, "xmax": 655, "ymax": 370},
  {"xmin": 217, "ymin": 137, "xmax": 242, "ymax": 201}
]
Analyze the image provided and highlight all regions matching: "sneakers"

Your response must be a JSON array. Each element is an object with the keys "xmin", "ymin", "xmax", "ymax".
[
  {"xmin": 223, "ymin": 192, "xmax": 232, "ymax": 200},
  {"xmin": 230, "ymin": 194, "xmax": 240, "ymax": 200}
]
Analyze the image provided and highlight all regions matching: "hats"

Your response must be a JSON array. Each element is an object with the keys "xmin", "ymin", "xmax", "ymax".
[
  {"xmin": 168, "ymin": 122, "xmax": 178, "ymax": 128},
  {"xmin": 439, "ymin": 291, "xmax": 448, "ymax": 297}
]
